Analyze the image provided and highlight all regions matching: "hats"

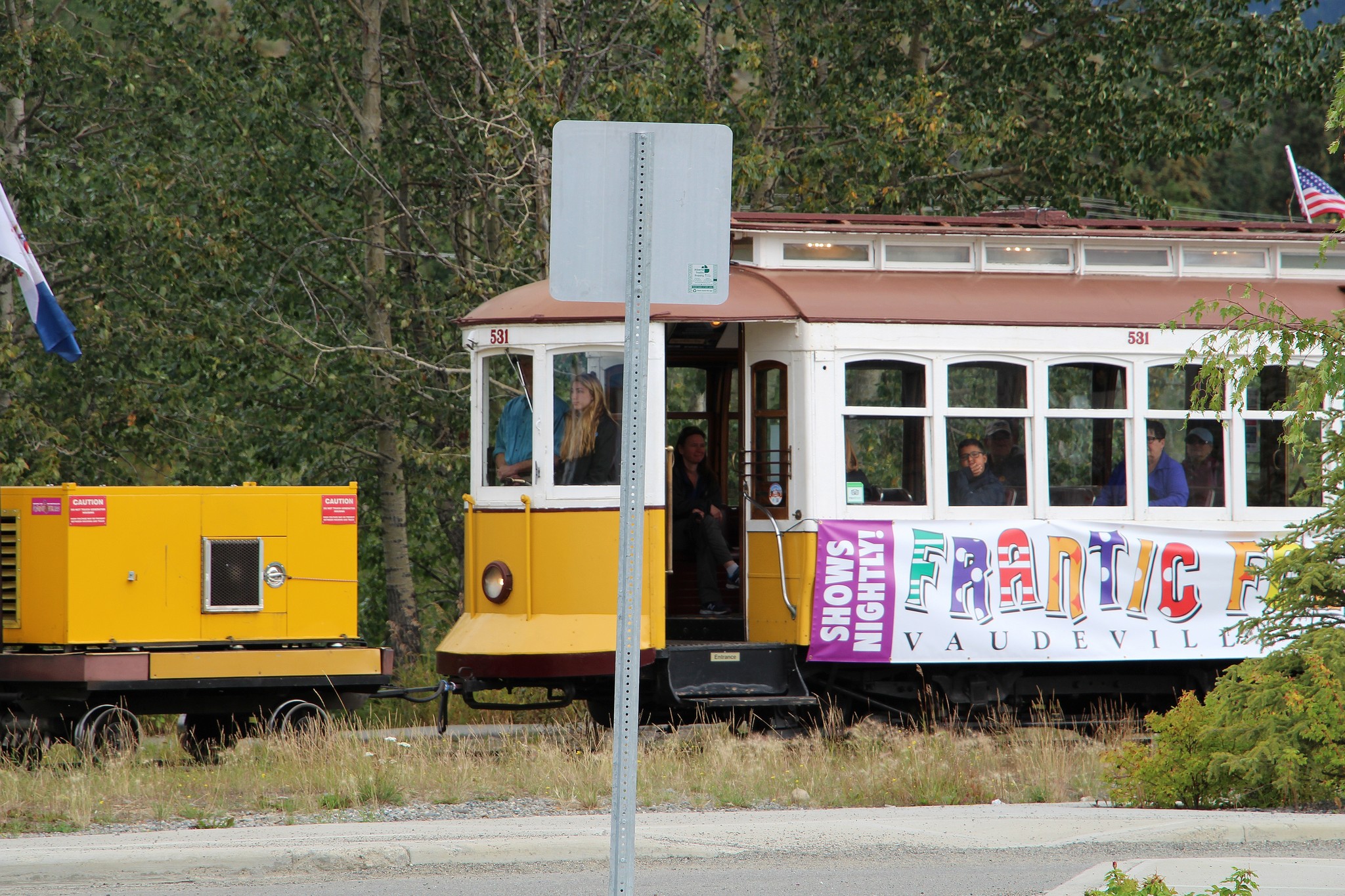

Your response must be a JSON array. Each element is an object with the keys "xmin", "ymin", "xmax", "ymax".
[
  {"xmin": 985, "ymin": 419, "xmax": 1012, "ymax": 437},
  {"xmin": 1185, "ymin": 427, "xmax": 1214, "ymax": 443}
]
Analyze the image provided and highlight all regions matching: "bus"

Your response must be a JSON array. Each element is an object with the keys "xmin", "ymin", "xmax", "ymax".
[{"xmin": 433, "ymin": 202, "xmax": 1345, "ymax": 730}]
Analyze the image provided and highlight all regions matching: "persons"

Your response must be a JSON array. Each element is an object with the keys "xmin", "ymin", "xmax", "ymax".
[
  {"xmin": 948, "ymin": 437, "xmax": 1006, "ymax": 506},
  {"xmin": 560, "ymin": 373, "xmax": 620, "ymax": 485},
  {"xmin": 845, "ymin": 432, "xmax": 876, "ymax": 501},
  {"xmin": 672, "ymin": 425, "xmax": 739, "ymax": 614},
  {"xmin": 1094, "ymin": 420, "xmax": 1189, "ymax": 506},
  {"xmin": 492, "ymin": 354, "xmax": 569, "ymax": 486},
  {"xmin": 984, "ymin": 419, "xmax": 1026, "ymax": 486},
  {"xmin": 1181, "ymin": 428, "xmax": 1225, "ymax": 507}
]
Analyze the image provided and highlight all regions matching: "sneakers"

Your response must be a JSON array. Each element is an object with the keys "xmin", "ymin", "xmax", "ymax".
[
  {"xmin": 726, "ymin": 566, "xmax": 740, "ymax": 588},
  {"xmin": 699, "ymin": 603, "xmax": 727, "ymax": 614}
]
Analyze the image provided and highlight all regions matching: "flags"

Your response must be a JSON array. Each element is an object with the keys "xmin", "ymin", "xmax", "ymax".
[
  {"xmin": 0, "ymin": 182, "xmax": 81, "ymax": 363},
  {"xmin": 1289, "ymin": 162, "xmax": 1345, "ymax": 219}
]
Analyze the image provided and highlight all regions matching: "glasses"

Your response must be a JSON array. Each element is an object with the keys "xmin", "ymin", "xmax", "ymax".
[
  {"xmin": 959, "ymin": 452, "xmax": 984, "ymax": 461},
  {"xmin": 1147, "ymin": 436, "xmax": 1161, "ymax": 444}
]
